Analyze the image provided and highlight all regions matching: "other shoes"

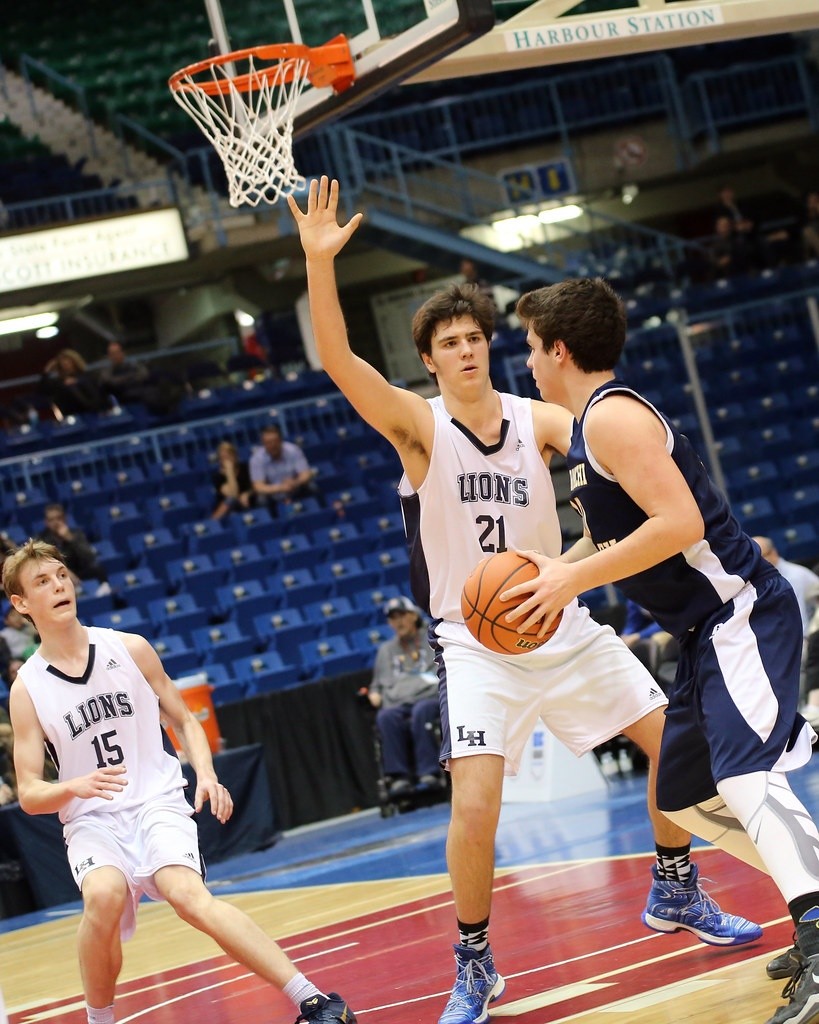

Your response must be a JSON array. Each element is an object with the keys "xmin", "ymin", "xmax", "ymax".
[
  {"xmin": 416, "ymin": 775, "xmax": 437, "ymax": 790},
  {"xmin": 801, "ymin": 710, "xmax": 819, "ymax": 727},
  {"xmin": 600, "ymin": 749, "xmax": 633, "ymax": 776},
  {"xmin": 391, "ymin": 780, "xmax": 411, "ymax": 795}
]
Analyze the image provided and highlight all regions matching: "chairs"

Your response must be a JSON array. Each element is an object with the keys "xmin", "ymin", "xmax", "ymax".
[
  {"xmin": 0, "ymin": 345, "xmax": 430, "ymax": 720},
  {"xmin": 490, "ymin": 238, "xmax": 819, "ymax": 568},
  {"xmin": 0, "ymin": 0, "xmax": 638, "ymax": 210}
]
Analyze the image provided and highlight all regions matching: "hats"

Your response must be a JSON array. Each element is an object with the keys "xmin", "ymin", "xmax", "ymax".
[{"xmin": 384, "ymin": 597, "xmax": 414, "ymax": 617}]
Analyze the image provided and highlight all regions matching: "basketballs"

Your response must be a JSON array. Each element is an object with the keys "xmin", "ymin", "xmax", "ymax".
[{"xmin": 460, "ymin": 550, "xmax": 566, "ymax": 657}]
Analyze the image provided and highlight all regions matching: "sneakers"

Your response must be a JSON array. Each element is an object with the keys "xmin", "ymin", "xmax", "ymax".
[
  {"xmin": 641, "ymin": 862, "xmax": 762, "ymax": 947},
  {"xmin": 295, "ymin": 993, "xmax": 358, "ymax": 1024},
  {"xmin": 766, "ymin": 940, "xmax": 806, "ymax": 978},
  {"xmin": 764, "ymin": 953, "xmax": 819, "ymax": 1024},
  {"xmin": 437, "ymin": 943, "xmax": 505, "ymax": 1024}
]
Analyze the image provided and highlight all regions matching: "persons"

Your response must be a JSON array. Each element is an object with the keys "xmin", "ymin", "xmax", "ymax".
[
  {"xmin": 2, "ymin": 328, "xmax": 359, "ymax": 1024},
  {"xmin": 366, "ymin": 593, "xmax": 440, "ymax": 795},
  {"xmin": 707, "ymin": 172, "xmax": 819, "ymax": 271},
  {"xmin": 283, "ymin": 171, "xmax": 765, "ymax": 951},
  {"xmin": 512, "ymin": 273, "xmax": 818, "ymax": 951}
]
